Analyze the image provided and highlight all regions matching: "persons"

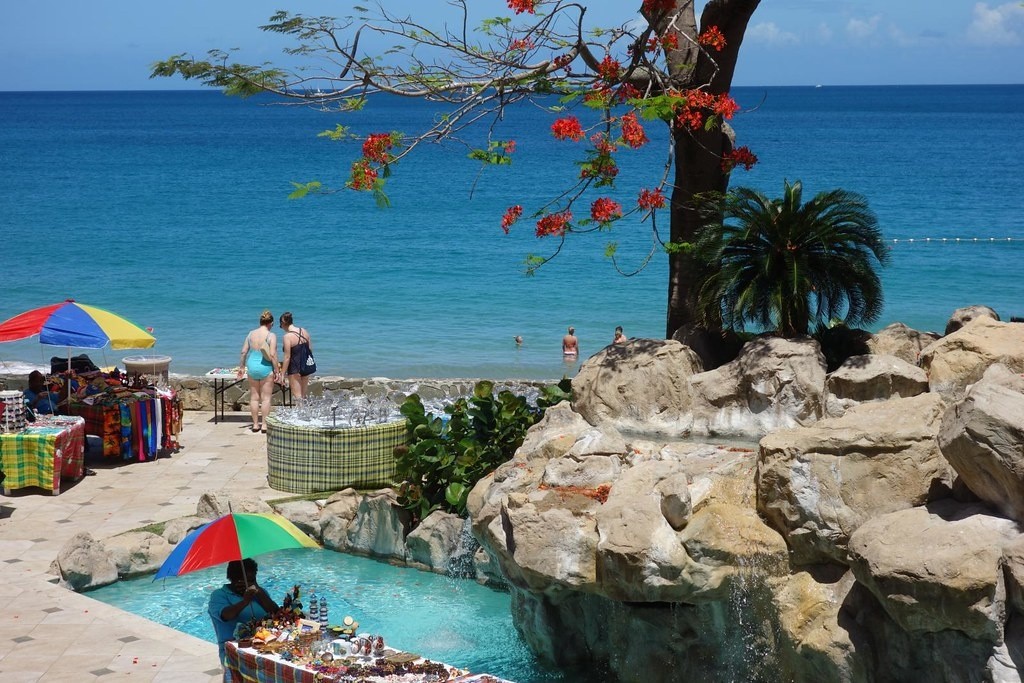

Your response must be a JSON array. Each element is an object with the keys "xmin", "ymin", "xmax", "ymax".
[
  {"xmin": 278, "ymin": 311, "xmax": 311, "ymax": 407},
  {"xmin": 24, "ymin": 369, "xmax": 97, "ymax": 475},
  {"xmin": 613, "ymin": 325, "xmax": 628, "ymax": 345},
  {"xmin": 513, "ymin": 336, "xmax": 523, "ymax": 345},
  {"xmin": 207, "ymin": 557, "xmax": 280, "ymax": 668},
  {"xmin": 561, "ymin": 326, "xmax": 580, "ymax": 361},
  {"xmin": 237, "ymin": 311, "xmax": 279, "ymax": 433}
]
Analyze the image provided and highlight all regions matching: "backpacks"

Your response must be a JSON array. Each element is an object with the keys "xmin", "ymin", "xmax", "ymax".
[{"xmin": 292, "ymin": 328, "xmax": 316, "ymax": 376}]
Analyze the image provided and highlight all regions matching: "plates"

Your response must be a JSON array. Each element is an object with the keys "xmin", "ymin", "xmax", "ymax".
[{"xmin": 385, "ymin": 652, "xmax": 421, "ymax": 663}]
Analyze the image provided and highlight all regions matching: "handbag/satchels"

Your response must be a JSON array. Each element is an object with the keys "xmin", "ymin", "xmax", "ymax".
[{"xmin": 260, "ymin": 333, "xmax": 272, "ymax": 361}]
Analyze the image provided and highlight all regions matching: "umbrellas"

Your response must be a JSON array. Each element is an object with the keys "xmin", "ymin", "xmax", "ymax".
[
  {"xmin": 0, "ymin": 298, "xmax": 156, "ymax": 417},
  {"xmin": 151, "ymin": 500, "xmax": 321, "ymax": 589}
]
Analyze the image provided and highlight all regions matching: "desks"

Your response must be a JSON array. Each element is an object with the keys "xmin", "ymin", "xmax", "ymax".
[
  {"xmin": 0, "ymin": 414, "xmax": 87, "ymax": 497},
  {"xmin": 223, "ymin": 633, "xmax": 517, "ymax": 683},
  {"xmin": 59, "ymin": 388, "xmax": 185, "ymax": 467},
  {"xmin": 204, "ymin": 367, "xmax": 292, "ymax": 426}
]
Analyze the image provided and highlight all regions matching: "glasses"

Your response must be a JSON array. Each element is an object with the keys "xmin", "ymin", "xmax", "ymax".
[{"xmin": 0, "ymin": 394, "xmax": 27, "ymax": 432}]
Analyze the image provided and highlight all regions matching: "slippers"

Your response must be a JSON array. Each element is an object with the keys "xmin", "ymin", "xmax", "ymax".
[
  {"xmin": 262, "ymin": 430, "xmax": 266, "ymax": 432},
  {"xmin": 253, "ymin": 427, "xmax": 261, "ymax": 432}
]
